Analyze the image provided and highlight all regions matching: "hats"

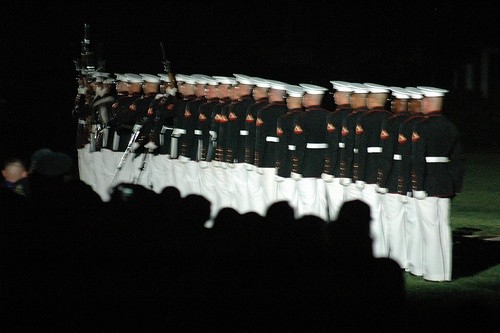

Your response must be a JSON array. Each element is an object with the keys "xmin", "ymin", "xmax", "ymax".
[
  {"xmin": 416, "ymin": 86, "xmax": 448, "ymax": 98},
  {"xmin": 405, "ymin": 86, "xmax": 424, "ymax": 100},
  {"xmin": 348, "ymin": 82, "xmax": 369, "ymax": 94},
  {"xmin": 330, "ymin": 80, "xmax": 353, "ymax": 92},
  {"xmin": 76, "ymin": 69, "xmax": 284, "ymax": 91},
  {"xmin": 298, "ymin": 83, "xmax": 328, "ymax": 95},
  {"xmin": 389, "ymin": 86, "xmax": 411, "ymax": 99},
  {"xmin": 363, "ymin": 82, "xmax": 390, "ymax": 94},
  {"xmin": 283, "ymin": 85, "xmax": 305, "ymax": 97}
]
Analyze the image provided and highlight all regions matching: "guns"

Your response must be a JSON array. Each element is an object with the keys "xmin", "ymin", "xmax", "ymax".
[
  {"xmin": 80, "ymin": 23, "xmax": 95, "ymax": 68},
  {"xmin": 72, "ymin": 58, "xmax": 85, "ymax": 149},
  {"xmin": 110, "ymin": 131, "xmax": 137, "ymax": 185},
  {"xmin": 137, "ymin": 148, "xmax": 148, "ymax": 182},
  {"xmin": 160, "ymin": 41, "xmax": 177, "ymax": 88}
]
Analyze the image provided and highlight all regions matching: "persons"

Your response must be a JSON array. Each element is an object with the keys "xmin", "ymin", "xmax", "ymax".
[
  {"xmin": 72, "ymin": 68, "xmax": 463, "ymax": 282},
  {"xmin": 0, "ymin": 140, "xmax": 408, "ymax": 333}
]
